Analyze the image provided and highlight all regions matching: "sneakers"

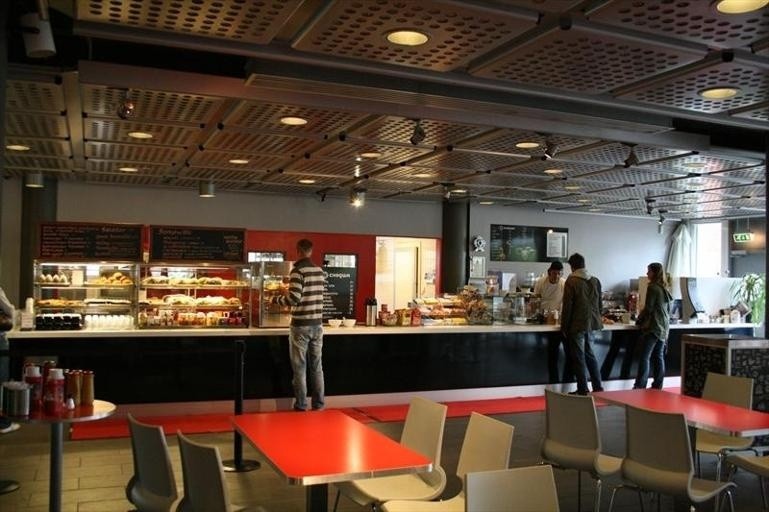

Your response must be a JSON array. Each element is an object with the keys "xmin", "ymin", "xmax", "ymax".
[{"xmin": 0, "ymin": 422, "xmax": 20, "ymax": 433}]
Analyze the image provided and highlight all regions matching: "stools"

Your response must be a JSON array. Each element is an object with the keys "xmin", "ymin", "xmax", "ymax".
[{"xmin": 718, "ymin": 451, "xmax": 769, "ymax": 511}]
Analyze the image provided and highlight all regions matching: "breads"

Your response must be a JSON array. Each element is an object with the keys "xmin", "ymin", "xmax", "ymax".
[
  {"xmin": 38, "ymin": 274, "xmax": 68, "ymax": 286},
  {"xmin": 142, "ymin": 275, "xmax": 247, "ymax": 289},
  {"xmin": 92, "ymin": 272, "xmax": 133, "ymax": 284},
  {"xmin": 143, "ymin": 293, "xmax": 242, "ymax": 308}
]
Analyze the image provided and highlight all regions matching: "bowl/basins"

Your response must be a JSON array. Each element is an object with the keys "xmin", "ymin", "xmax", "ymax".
[
  {"xmin": 328, "ymin": 319, "xmax": 342, "ymax": 328},
  {"xmin": 342, "ymin": 320, "xmax": 357, "ymax": 328}
]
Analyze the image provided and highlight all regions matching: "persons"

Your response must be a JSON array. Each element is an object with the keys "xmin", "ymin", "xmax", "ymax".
[
  {"xmin": 0, "ymin": 286, "xmax": 14, "ymax": 381},
  {"xmin": 267, "ymin": 239, "xmax": 327, "ymax": 410},
  {"xmin": 527, "ymin": 260, "xmax": 566, "ymax": 311},
  {"xmin": 561, "ymin": 252, "xmax": 604, "ymax": 396},
  {"xmin": 633, "ymin": 264, "xmax": 674, "ymax": 391}
]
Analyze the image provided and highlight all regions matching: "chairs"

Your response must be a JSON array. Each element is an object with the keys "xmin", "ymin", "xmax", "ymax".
[
  {"xmin": 332, "ymin": 392, "xmax": 451, "ymax": 511},
  {"xmin": 536, "ymin": 387, "xmax": 625, "ymax": 512},
  {"xmin": 692, "ymin": 371, "xmax": 758, "ymax": 481},
  {"xmin": 174, "ymin": 425, "xmax": 243, "ymax": 512},
  {"xmin": 122, "ymin": 409, "xmax": 179, "ymax": 511},
  {"xmin": 459, "ymin": 463, "xmax": 563, "ymax": 512},
  {"xmin": 606, "ymin": 401, "xmax": 741, "ymax": 510},
  {"xmin": 378, "ymin": 408, "xmax": 518, "ymax": 511}
]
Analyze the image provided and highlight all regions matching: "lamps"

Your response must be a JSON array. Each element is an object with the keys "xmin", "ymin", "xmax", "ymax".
[
  {"xmin": 409, "ymin": 130, "xmax": 426, "ymax": 145},
  {"xmin": 197, "ymin": 180, "xmax": 216, "ymax": 199},
  {"xmin": 625, "ymin": 155, "xmax": 640, "ymax": 166},
  {"xmin": 113, "ymin": 89, "xmax": 136, "ymax": 122},
  {"xmin": 16, "ymin": 0, "xmax": 58, "ymax": 61},
  {"xmin": 343, "ymin": 115, "xmax": 674, "ymax": 226},
  {"xmin": 544, "ymin": 142, "xmax": 559, "ymax": 157},
  {"xmin": 24, "ymin": 169, "xmax": 45, "ymax": 190}
]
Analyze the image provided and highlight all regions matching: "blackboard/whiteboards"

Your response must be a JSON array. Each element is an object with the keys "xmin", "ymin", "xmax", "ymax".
[
  {"xmin": 35, "ymin": 220, "xmax": 144, "ymax": 263},
  {"xmin": 149, "ymin": 224, "xmax": 248, "ymax": 266}
]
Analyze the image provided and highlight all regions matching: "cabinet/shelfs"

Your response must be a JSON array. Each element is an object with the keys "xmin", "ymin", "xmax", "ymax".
[
  {"xmin": 29, "ymin": 257, "xmax": 297, "ymax": 330},
  {"xmin": 412, "ymin": 297, "xmax": 467, "ymax": 325}
]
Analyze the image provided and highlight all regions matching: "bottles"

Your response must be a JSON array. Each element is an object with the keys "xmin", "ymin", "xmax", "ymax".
[
  {"xmin": 146, "ymin": 311, "xmax": 248, "ymax": 327},
  {"xmin": 24, "ymin": 359, "xmax": 95, "ymax": 418}
]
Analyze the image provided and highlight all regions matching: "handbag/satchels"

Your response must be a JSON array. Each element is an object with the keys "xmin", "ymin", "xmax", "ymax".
[{"xmin": 0, "ymin": 312, "xmax": 12, "ymax": 331}]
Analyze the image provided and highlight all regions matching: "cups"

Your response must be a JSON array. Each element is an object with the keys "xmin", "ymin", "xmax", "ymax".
[
  {"xmin": 7, "ymin": 387, "xmax": 32, "ymax": 417},
  {"xmin": 622, "ymin": 313, "xmax": 631, "ymax": 324},
  {"xmin": 85, "ymin": 314, "xmax": 132, "ymax": 329}
]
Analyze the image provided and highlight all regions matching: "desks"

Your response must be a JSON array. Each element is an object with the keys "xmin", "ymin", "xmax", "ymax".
[
  {"xmin": 229, "ymin": 405, "xmax": 439, "ymax": 511},
  {"xmin": 586, "ymin": 387, "xmax": 768, "ymax": 473},
  {"xmin": 1, "ymin": 396, "xmax": 118, "ymax": 512}
]
